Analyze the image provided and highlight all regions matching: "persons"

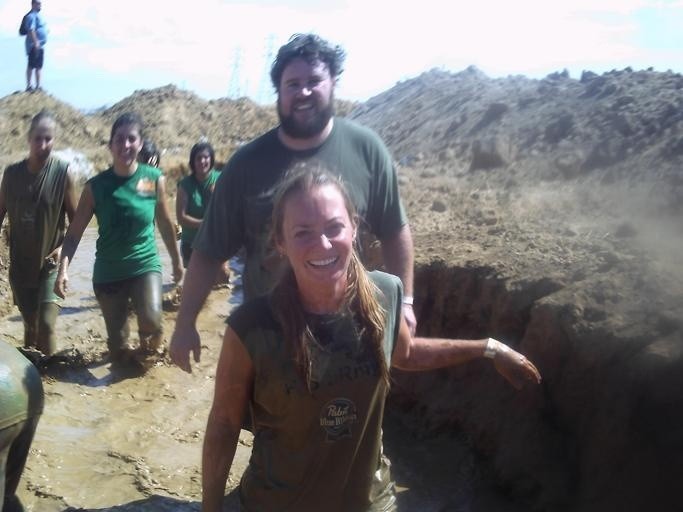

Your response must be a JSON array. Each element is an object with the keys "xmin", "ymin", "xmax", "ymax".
[
  {"xmin": 168, "ymin": 32, "xmax": 417, "ymax": 374},
  {"xmin": 197, "ymin": 157, "xmax": 543, "ymax": 511},
  {"xmin": 0, "ymin": 338, "xmax": 44, "ymax": 512},
  {"xmin": 52, "ymin": 111, "xmax": 185, "ymax": 355},
  {"xmin": 18, "ymin": 0, "xmax": 47, "ymax": 90},
  {"xmin": 0, "ymin": 110, "xmax": 79, "ymax": 355},
  {"xmin": 136, "ymin": 140, "xmax": 162, "ymax": 167},
  {"xmin": 175, "ymin": 136, "xmax": 231, "ymax": 285}
]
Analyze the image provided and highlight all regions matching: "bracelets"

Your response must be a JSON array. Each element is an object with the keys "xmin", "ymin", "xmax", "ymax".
[
  {"xmin": 481, "ymin": 335, "xmax": 499, "ymax": 361},
  {"xmin": 401, "ymin": 295, "xmax": 414, "ymax": 305}
]
